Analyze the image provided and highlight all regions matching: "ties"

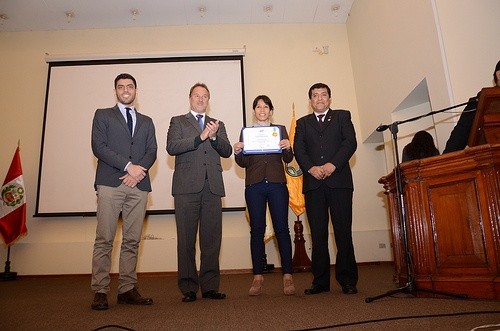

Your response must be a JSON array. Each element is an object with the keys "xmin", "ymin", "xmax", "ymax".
[
  {"xmin": 316, "ymin": 114, "xmax": 324, "ymax": 127},
  {"xmin": 196, "ymin": 114, "xmax": 207, "ymax": 132},
  {"xmin": 122, "ymin": 106, "xmax": 134, "ymax": 140}
]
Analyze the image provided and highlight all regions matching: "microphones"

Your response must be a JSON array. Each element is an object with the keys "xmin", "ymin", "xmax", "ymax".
[{"xmin": 376, "ymin": 125, "xmax": 389, "ymax": 132}]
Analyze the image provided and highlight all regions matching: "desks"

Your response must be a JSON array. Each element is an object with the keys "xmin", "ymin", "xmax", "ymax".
[{"xmin": 378, "ymin": 142, "xmax": 500, "ymax": 301}]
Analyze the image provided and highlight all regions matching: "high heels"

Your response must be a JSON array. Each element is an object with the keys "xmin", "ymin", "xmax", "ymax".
[{"xmin": 247, "ymin": 273, "xmax": 297, "ymax": 297}]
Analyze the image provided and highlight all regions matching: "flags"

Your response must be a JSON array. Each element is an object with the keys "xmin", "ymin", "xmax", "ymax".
[{"xmin": 0, "ymin": 146, "xmax": 27, "ymax": 248}]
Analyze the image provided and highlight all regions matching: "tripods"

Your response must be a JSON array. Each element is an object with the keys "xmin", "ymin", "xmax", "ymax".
[{"xmin": 365, "ymin": 134, "xmax": 469, "ymax": 304}]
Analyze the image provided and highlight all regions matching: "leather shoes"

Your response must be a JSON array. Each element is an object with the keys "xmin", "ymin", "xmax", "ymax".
[
  {"xmin": 89, "ymin": 287, "xmax": 155, "ymax": 310},
  {"xmin": 304, "ymin": 283, "xmax": 359, "ymax": 296},
  {"xmin": 181, "ymin": 289, "xmax": 226, "ymax": 303}
]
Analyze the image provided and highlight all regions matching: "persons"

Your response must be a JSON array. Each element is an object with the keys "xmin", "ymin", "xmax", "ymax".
[
  {"xmin": 166, "ymin": 82, "xmax": 232, "ymax": 302},
  {"xmin": 91, "ymin": 74, "xmax": 157, "ymax": 310},
  {"xmin": 402, "ymin": 130, "xmax": 440, "ymax": 163},
  {"xmin": 233, "ymin": 95, "xmax": 295, "ymax": 296},
  {"xmin": 442, "ymin": 61, "xmax": 500, "ymax": 154},
  {"xmin": 294, "ymin": 83, "xmax": 358, "ymax": 294}
]
